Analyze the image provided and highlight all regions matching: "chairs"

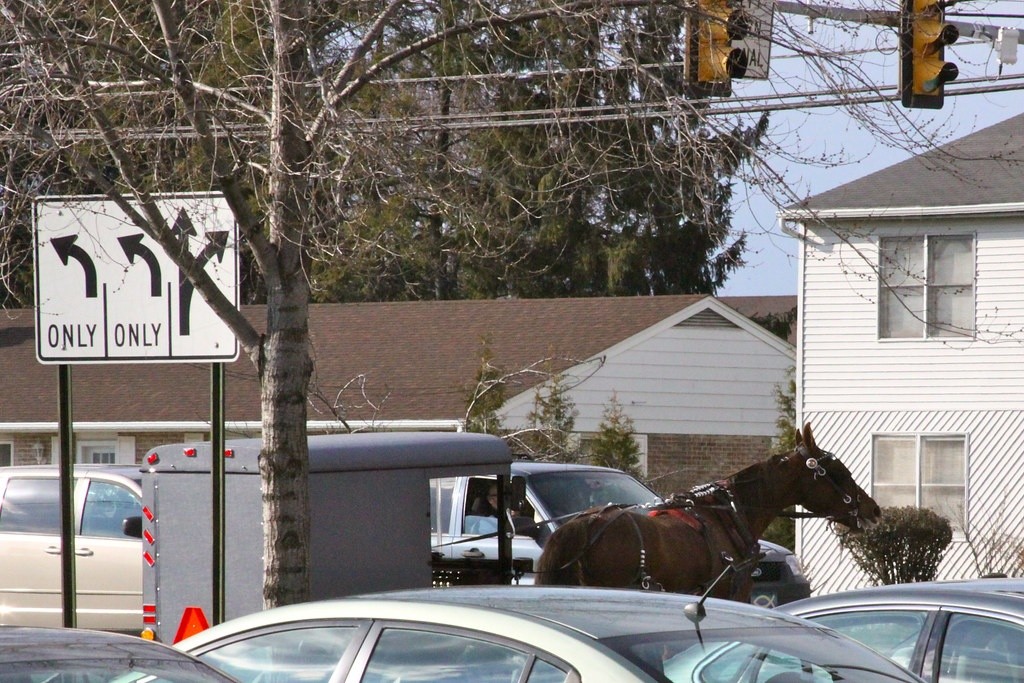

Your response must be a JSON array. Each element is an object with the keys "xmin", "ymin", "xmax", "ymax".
[{"xmin": 469, "ymin": 488, "xmax": 490, "ymax": 514}]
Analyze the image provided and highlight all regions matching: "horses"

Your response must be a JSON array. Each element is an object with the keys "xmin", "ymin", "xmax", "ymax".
[{"xmin": 535, "ymin": 422, "xmax": 882, "ymax": 606}]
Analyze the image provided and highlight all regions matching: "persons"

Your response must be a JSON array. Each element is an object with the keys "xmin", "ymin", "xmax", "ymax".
[{"xmin": 483, "ymin": 483, "xmax": 497, "ymax": 517}]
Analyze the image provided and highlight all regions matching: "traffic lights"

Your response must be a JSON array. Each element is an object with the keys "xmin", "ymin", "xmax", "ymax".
[
  {"xmin": 685, "ymin": 0, "xmax": 748, "ymax": 98},
  {"xmin": 898, "ymin": 0, "xmax": 961, "ymax": 109}
]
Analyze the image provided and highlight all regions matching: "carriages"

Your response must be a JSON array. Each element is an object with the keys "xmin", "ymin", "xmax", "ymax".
[{"xmin": 140, "ymin": 422, "xmax": 885, "ymax": 649}]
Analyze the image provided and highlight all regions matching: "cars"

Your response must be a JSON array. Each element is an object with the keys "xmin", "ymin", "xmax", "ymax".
[
  {"xmin": 1, "ymin": 463, "xmax": 143, "ymax": 630},
  {"xmin": 174, "ymin": 581, "xmax": 931, "ymax": 683},
  {"xmin": 426, "ymin": 463, "xmax": 813, "ymax": 610},
  {"xmin": 664, "ymin": 575, "xmax": 1024, "ymax": 683},
  {"xmin": 2, "ymin": 628, "xmax": 238, "ymax": 683}
]
